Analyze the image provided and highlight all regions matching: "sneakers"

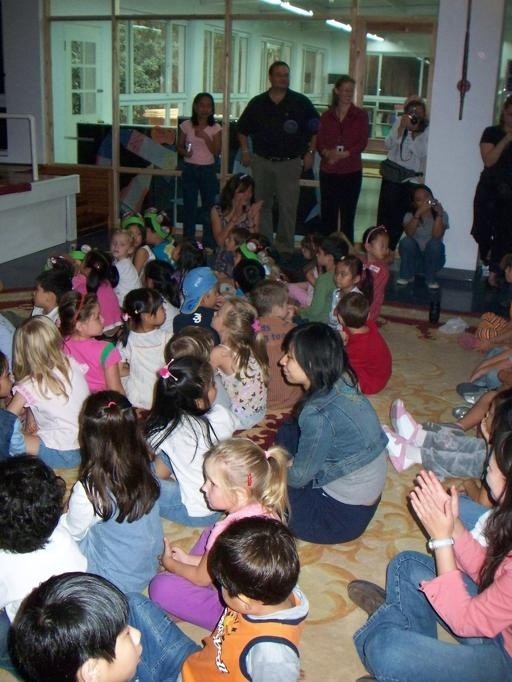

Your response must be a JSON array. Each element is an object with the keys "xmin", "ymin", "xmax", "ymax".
[
  {"xmin": 346, "ymin": 578, "xmax": 388, "ymax": 621},
  {"xmin": 388, "ymin": 396, "xmax": 422, "ymax": 446},
  {"xmin": 380, "ymin": 423, "xmax": 415, "ymax": 475},
  {"xmin": 424, "ymin": 276, "xmax": 441, "ymax": 290},
  {"xmin": 451, "ymin": 380, "xmax": 491, "ymax": 421},
  {"xmin": 422, "ymin": 421, "xmax": 467, "ymax": 438},
  {"xmin": 396, "ymin": 275, "xmax": 416, "ymax": 287}
]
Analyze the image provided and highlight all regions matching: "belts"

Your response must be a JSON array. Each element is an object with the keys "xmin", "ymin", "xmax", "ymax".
[{"xmin": 251, "ymin": 150, "xmax": 301, "ymax": 163}]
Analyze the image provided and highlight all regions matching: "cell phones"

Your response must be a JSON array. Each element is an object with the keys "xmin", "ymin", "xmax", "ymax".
[{"xmin": 337, "ymin": 145, "xmax": 344, "ymax": 153}]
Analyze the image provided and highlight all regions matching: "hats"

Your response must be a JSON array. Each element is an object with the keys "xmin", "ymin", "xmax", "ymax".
[{"xmin": 179, "ymin": 266, "xmax": 219, "ymax": 315}]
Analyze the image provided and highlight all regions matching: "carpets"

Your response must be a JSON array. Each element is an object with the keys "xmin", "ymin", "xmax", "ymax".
[{"xmin": 0, "ymin": 282, "xmax": 493, "ymax": 680}]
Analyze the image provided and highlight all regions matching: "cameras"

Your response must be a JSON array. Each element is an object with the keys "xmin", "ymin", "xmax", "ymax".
[
  {"xmin": 427, "ymin": 198, "xmax": 438, "ymax": 206},
  {"xmin": 185, "ymin": 142, "xmax": 192, "ymax": 152},
  {"xmin": 407, "ymin": 103, "xmax": 419, "ymax": 124}
]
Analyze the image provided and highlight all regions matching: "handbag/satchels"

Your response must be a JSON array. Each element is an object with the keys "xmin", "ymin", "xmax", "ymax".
[{"xmin": 379, "ymin": 159, "xmax": 424, "ymax": 183}]
